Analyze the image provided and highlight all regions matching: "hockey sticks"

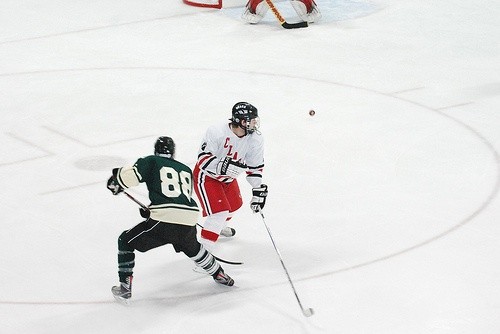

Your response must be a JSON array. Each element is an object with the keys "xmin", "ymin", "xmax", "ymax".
[
  {"xmin": 119, "ymin": 186, "xmax": 244, "ymax": 267},
  {"xmin": 254, "ymin": 202, "xmax": 316, "ymax": 320},
  {"xmin": 266, "ymin": 0, "xmax": 308, "ymax": 29}
]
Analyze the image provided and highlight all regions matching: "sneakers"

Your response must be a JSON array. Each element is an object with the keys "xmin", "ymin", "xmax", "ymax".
[
  {"xmin": 111, "ymin": 272, "xmax": 133, "ymax": 305},
  {"xmin": 211, "ymin": 266, "xmax": 238, "ymax": 288}
]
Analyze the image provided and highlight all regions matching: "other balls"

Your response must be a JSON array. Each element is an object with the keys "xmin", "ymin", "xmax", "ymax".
[{"xmin": 308, "ymin": 109, "xmax": 316, "ymax": 117}]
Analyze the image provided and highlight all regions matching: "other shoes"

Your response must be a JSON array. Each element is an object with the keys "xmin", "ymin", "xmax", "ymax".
[{"xmin": 220, "ymin": 227, "xmax": 236, "ymax": 238}]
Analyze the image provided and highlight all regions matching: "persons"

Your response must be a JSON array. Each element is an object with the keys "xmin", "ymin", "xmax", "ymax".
[
  {"xmin": 241, "ymin": 0, "xmax": 320, "ymax": 24},
  {"xmin": 193, "ymin": 102, "xmax": 268, "ymax": 275},
  {"xmin": 107, "ymin": 136, "xmax": 239, "ymax": 305}
]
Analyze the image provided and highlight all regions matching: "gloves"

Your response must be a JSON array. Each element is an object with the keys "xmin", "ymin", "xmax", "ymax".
[
  {"xmin": 250, "ymin": 184, "xmax": 268, "ymax": 213},
  {"xmin": 217, "ymin": 156, "xmax": 247, "ymax": 178},
  {"xmin": 107, "ymin": 168, "xmax": 124, "ymax": 195},
  {"xmin": 139, "ymin": 206, "xmax": 150, "ymax": 218}
]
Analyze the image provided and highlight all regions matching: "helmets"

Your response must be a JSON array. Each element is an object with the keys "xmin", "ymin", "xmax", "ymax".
[
  {"xmin": 154, "ymin": 136, "xmax": 175, "ymax": 159},
  {"xmin": 229, "ymin": 102, "xmax": 257, "ymax": 134}
]
